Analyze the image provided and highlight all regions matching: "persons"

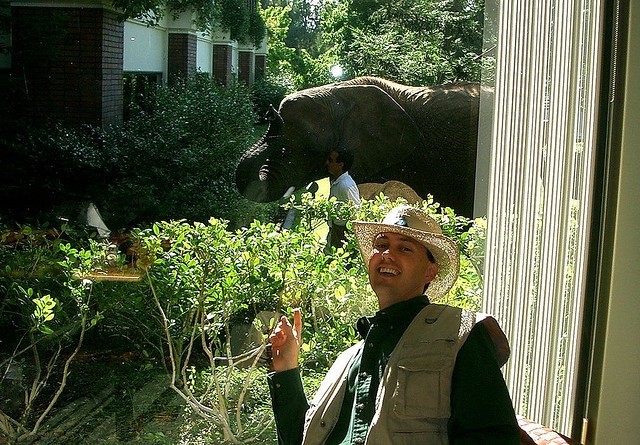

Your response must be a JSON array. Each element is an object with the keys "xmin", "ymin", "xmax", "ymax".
[
  {"xmin": 325, "ymin": 146, "xmax": 362, "ymax": 248},
  {"xmin": 266, "ymin": 205, "xmax": 522, "ymax": 445}
]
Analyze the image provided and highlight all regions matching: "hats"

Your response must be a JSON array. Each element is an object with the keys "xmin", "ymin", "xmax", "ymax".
[
  {"xmin": 336, "ymin": 144, "xmax": 354, "ymax": 169},
  {"xmin": 350, "ymin": 205, "xmax": 460, "ymax": 302}
]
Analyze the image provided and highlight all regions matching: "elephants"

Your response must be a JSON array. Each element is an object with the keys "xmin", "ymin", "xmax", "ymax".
[{"xmin": 236, "ymin": 76, "xmax": 494, "ymax": 256}]
